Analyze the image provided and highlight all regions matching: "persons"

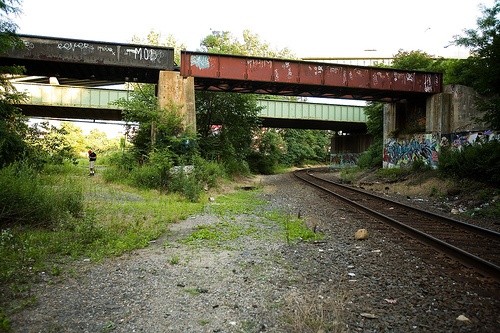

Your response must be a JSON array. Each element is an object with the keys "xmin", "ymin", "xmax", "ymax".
[{"xmin": 88, "ymin": 150, "xmax": 97, "ymax": 176}]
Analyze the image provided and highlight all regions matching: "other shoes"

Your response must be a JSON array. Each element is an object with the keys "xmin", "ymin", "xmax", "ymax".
[{"xmin": 88, "ymin": 173, "xmax": 94, "ymax": 177}]
